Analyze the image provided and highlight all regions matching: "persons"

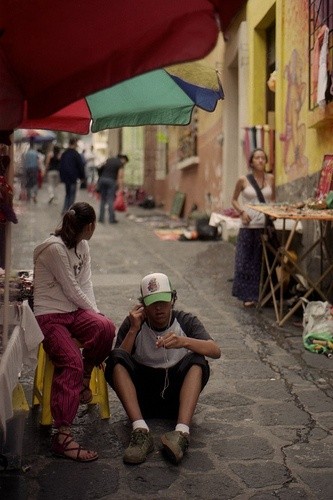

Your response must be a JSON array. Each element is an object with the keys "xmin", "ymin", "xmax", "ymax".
[
  {"xmin": 103, "ymin": 272, "xmax": 221, "ymax": 465},
  {"xmin": 230, "ymin": 149, "xmax": 293, "ymax": 306},
  {"xmin": 0, "ymin": 137, "xmax": 224, "ymax": 241},
  {"xmin": 33, "ymin": 202, "xmax": 116, "ymax": 462}
]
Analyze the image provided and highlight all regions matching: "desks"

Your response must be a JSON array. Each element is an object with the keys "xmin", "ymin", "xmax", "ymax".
[
  {"xmin": 246, "ymin": 203, "xmax": 333, "ymax": 327},
  {"xmin": 0, "ymin": 268, "xmax": 44, "ymax": 440}
]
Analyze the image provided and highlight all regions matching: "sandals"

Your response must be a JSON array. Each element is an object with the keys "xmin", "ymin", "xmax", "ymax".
[
  {"xmin": 49, "ymin": 430, "xmax": 99, "ymax": 462},
  {"xmin": 79, "ymin": 369, "xmax": 93, "ymax": 404}
]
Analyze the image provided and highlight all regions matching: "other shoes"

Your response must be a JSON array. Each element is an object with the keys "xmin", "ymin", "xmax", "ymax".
[
  {"xmin": 243, "ymin": 300, "xmax": 256, "ymax": 307},
  {"xmin": 32, "ymin": 196, "xmax": 38, "ymax": 204},
  {"xmin": 98, "ymin": 219, "xmax": 104, "ymax": 225},
  {"xmin": 110, "ymin": 220, "xmax": 119, "ymax": 223},
  {"xmin": 48, "ymin": 195, "xmax": 54, "ymax": 204}
]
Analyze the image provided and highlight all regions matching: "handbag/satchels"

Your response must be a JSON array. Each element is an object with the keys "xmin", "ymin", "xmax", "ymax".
[
  {"xmin": 265, "ymin": 217, "xmax": 281, "ymax": 251},
  {"xmin": 299, "ymin": 296, "xmax": 333, "ymax": 336}
]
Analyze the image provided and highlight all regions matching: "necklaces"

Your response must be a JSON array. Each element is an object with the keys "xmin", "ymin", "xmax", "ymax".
[{"xmin": 259, "ymin": 174, "xmax": 264, "ymax": 189}]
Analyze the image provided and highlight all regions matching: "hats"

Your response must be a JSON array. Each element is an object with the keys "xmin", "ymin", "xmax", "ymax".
[{"xmin": 139, "ymin": 273, "xmax": 173, "ymax": 306}]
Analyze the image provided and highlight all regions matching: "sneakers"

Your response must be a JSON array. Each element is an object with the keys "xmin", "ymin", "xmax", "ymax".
[
  {"xmin": 123, "ymin": 427, "xmax": 155, "ymax": 464},
  {"xmin": 160, "ymin": 429, "xmax": 191, "ymax": 464}
]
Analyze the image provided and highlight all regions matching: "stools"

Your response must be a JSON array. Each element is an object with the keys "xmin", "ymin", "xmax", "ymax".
[
  {"xmin": 32, "ymin": 341, "xmax": 110, "ymax": 426},
  {"xmin": 3, "ymin": 382, "xmax": 29, "ymax": 468}
]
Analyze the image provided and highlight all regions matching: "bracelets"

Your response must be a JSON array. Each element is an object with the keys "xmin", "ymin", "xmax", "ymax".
[{"xmin": 240, "ymin": 211, "xmax": 244, "ymax": 215}]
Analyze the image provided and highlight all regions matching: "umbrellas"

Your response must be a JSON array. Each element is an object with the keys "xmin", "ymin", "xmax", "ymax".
[{"xmin": 0, "ymin": 0, "xmax": 248, "ymax": 142}]
